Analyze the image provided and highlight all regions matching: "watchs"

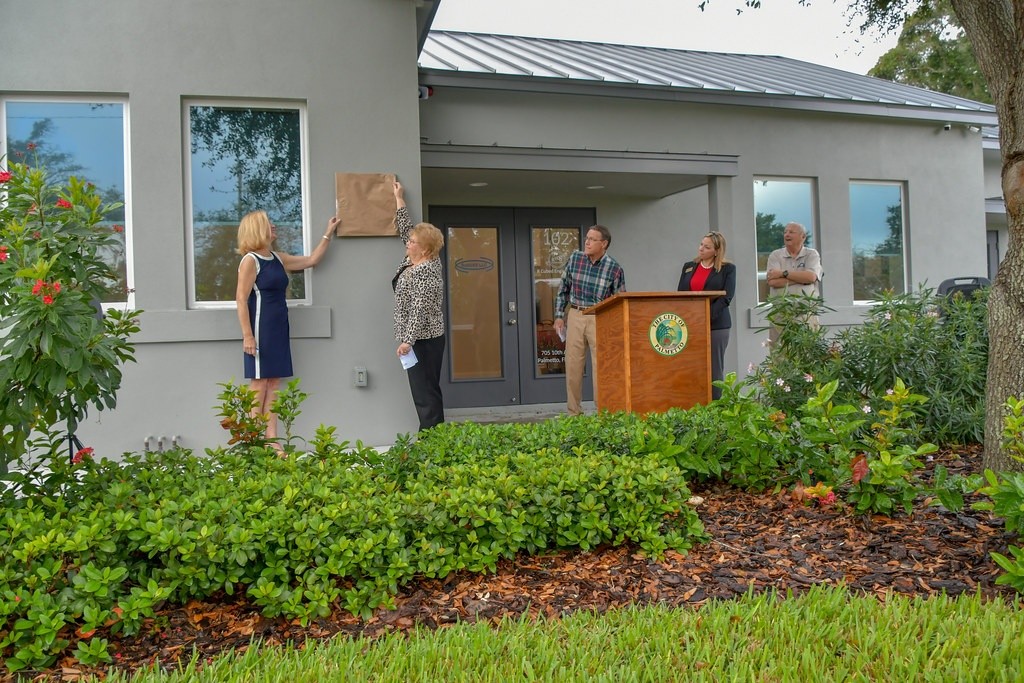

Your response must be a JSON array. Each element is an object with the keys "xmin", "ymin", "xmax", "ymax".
[{"xmin": 783, "ymin": 270, "xmax": 788, "ymax": 278}]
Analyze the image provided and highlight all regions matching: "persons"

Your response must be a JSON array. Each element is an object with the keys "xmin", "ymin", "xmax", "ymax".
[
  {"xmin": 392, "ymin": 182, "xmax": 445, "ymax": 443},
  {"xmin": 553, "ymin": 225, "xmax": 626, "ymax": 416},
  {"xmin": 236, "ymin": 211, "xmax": 341, "ymax": 460},
  {"xmin": 678, "ymin": 231, "xmax": 736, "ymax": 407},
  {"xmin": 766, "ymin": 223, "xmax": 824, "ymax": 354}
]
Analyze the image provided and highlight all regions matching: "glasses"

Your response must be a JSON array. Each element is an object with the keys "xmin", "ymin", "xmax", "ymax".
[
  {"xmin": 407, "ymin": 238, "xmax": 419, "ymax": 244},
  {"xmin": 585, "ymin": 237, "xmax": 602, "ymax": 243}
]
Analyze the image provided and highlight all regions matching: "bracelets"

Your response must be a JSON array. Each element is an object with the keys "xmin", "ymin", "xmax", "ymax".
[{"xmin": 322, "ymin": 235, "xmax": 330, "ymax": 241}]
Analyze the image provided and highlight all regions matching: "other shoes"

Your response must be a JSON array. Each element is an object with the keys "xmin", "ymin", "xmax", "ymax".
[{"xmin": 263, "ymin": 442, "xmax": 290, "ymax": 462}]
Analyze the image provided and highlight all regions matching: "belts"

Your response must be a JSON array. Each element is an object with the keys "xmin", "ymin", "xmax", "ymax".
[{"xmin": 570, "ymin": 305, "xmax": 590, "ymax": 312}]
